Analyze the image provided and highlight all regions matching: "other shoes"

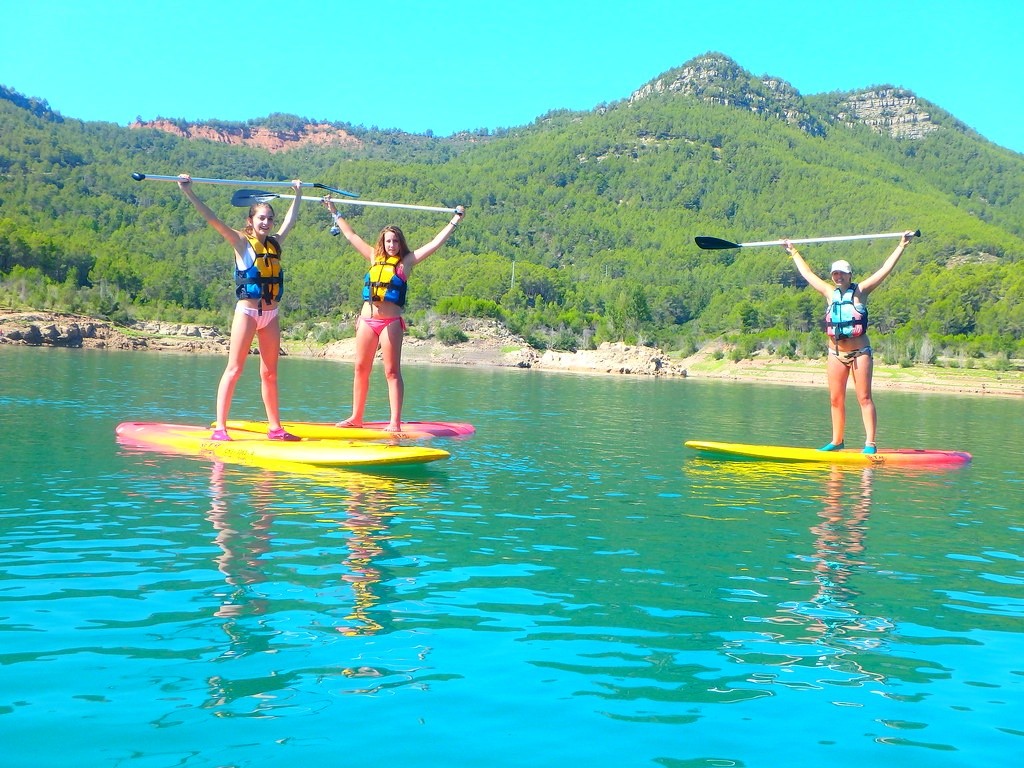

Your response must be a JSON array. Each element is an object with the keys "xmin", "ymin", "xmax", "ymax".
[
  {"xmin": 212, "ymin": 430, "xmax": 232, "ymax": 440},
  {"xmin": 822, "ymin": 440, "xmax": 844, "ymax": 450},
  {"xmin": 863, "ymin": 446, "xmax": 877, "ymax": 454},
  {"xmin": 268, "ymin": 427, "xmax": 301, "ymax": 440}
]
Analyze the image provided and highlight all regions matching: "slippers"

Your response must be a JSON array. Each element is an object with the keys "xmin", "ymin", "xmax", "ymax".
[{"xmin": 336, "ymin": 420, "xmax": 362, "ymax": 428}]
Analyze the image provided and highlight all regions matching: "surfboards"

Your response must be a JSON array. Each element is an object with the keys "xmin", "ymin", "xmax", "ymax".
[
  {"xmin": 116, "ymin": 422, "xmax": 451, "ymax": 467},
  {"xmin": 684, "ymin": 441, "xmax": 973, "ymax": 465},
  {"xmin": 211, "ymin": 419, "xmax": 476, "ymax": 439}
]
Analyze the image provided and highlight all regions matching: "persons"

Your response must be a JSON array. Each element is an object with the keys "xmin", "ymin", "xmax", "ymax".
[
  {"xmin": 322, "ymin": 193, "xmax": 467, "ymax": 433},
  {"xmin": 175, "ymin": 174, "xmax": 304, "ymax": 442},
  {"xmin": 780, "ymin": 228, "xmax": 916, "ymax": 455}
]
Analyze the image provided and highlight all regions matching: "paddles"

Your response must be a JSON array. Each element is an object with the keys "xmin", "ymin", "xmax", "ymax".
[
  {"xmin": 695, "ymin": 229, "xmax": 921, "ymax": 249},
  {"xmin": 132, "ymin": 172, "xmax": 361, "ymax": 198},
  {"xmin": 230, "ymin": 188, "xmax": 463, "ymax": 216}
]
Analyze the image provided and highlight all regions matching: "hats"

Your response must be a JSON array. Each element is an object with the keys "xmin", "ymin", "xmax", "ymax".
[{"xmin": 830, "ymin": 260, "xmax": 852, "ymax": 274}]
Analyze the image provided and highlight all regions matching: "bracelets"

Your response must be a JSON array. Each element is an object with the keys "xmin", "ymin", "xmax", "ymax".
[
  {"xmin": 450, "ymin": 220, "xmax": 460, "ymax": 228},
  {"xmin": 791, "ymin": 249, "xmax": 798, "ymax": 256}
]
